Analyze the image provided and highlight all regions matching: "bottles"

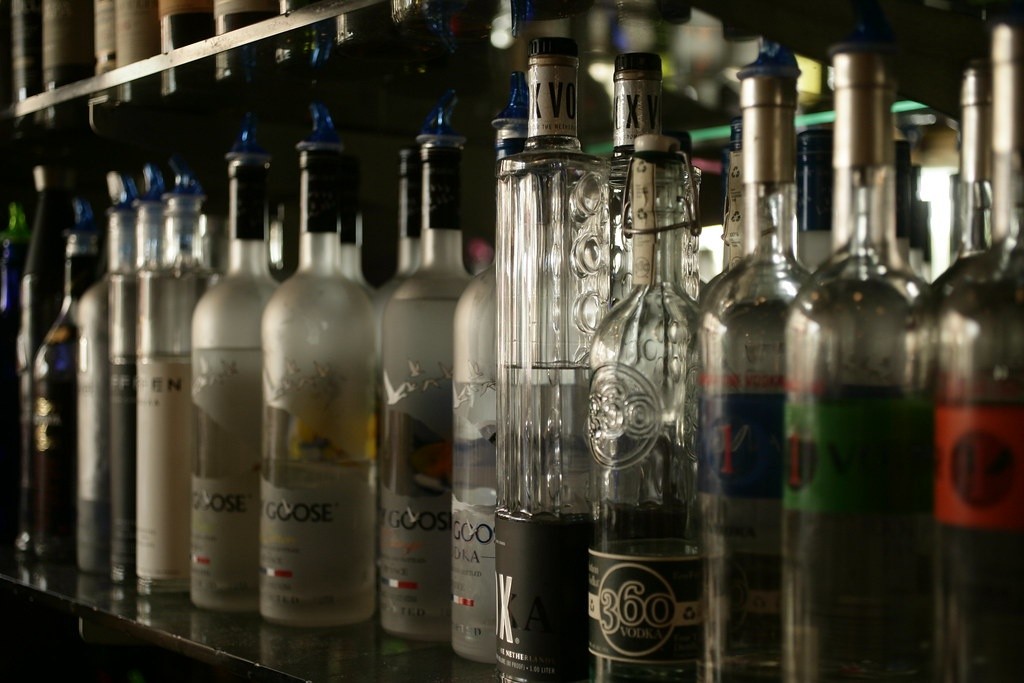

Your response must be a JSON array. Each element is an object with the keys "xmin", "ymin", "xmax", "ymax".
[{"xmin": 0, "ymin": 0, "xmax": 1024, "ymax": 683}]
